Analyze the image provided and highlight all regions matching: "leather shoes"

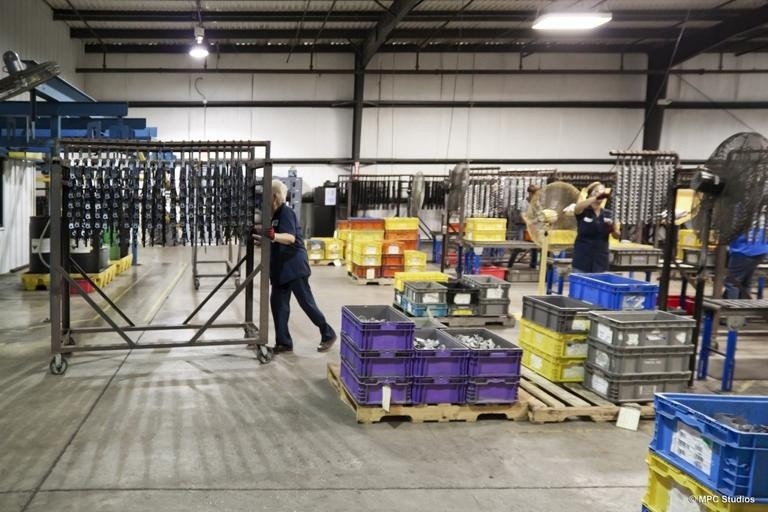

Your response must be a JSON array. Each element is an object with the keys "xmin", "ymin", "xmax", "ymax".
[
  {"xmin": 273, "ymin": 341, "xmax": 293, "ymax": 351},
  {"xmin": 315, "ymin": 334, "xmax": 338, "ymax": 350}
]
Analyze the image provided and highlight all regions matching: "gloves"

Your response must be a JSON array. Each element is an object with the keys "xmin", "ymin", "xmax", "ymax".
[{"xmin": 264, "ymin": 226, "xmax": 277, "ymax": 243}]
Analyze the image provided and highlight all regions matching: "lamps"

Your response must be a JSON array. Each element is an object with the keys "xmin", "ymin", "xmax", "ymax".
[
  {"xmin": 189, "ymin": 23, "xmax": 210, "ymax": 59},
  {"xmin": 531, "ymin": 0, "xmax": 613, "ymax": 32}
]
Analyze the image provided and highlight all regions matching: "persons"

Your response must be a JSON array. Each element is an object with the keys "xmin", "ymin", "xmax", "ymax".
[
  {"xmin": 723, "ymin": 193, "xmax": 768, "ymax": 300},
  {"xmin": 571, "ymin": 180, "xmax": 623, "ymax": 274},
  {"xmin": 247, "ymin": 177, "xmax": 338, "ymax": 354},
  {"xmin": 507, "ymin": 184, "xmax": 539, "ymax": 269}
]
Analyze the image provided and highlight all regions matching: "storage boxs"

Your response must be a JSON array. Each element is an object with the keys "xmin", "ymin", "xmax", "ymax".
[
  {"xmin": 521, "ymin": 271, "xmax": 768, "ymax": 511},
  {"xmin": 304, "ymin": 215, "xmax": 521, "ymax": 407}
]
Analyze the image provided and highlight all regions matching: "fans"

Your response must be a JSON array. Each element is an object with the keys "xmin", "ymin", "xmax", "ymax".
[
  {"xmin": 524, "ymin": 181, "xmax": 583, "ymax": 294},
  {"xmin": 0, "ymin": 50, "xmax": 61, "ymax": 102},
  {"xmin": 689, "ymin": 132, "xmax": 768, "ymax": 279}
]
